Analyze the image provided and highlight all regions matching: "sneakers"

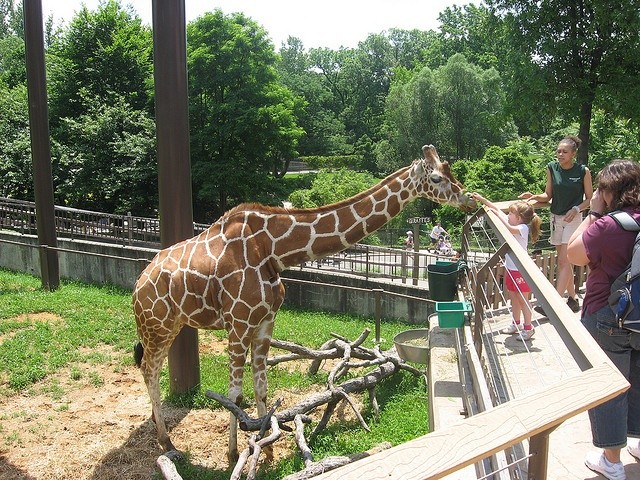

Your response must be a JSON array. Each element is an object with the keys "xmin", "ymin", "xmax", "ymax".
[
  {"xmin": 502, "ymin": 323, "xmax": 523, "ymax": 333},
  {"xmin": 534, "ymin": 306, "xmax": 547, "ymax": 317},
  {"xmin": 566, "ymin": 296, "xmax": 580, "ymax": 313},
  {"xmin": 585, "ymin": 449, "xmax": 626, "ymax": 480},
  {"xmin": 627, "ymin": 440, "xmax": 640, "ymax": 460},
  {"xmin": 516, "ymin": 327, "xmax": 535, "ymax": 341}
]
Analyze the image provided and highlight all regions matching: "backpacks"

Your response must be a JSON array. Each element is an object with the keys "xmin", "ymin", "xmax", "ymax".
[{"xmin": 607, "ymin": 210, "xmax": 640, "ymax": 334}]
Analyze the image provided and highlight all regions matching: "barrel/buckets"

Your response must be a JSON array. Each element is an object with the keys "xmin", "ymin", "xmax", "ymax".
[{"xmin": 427, "ymin": 264, "xmax": 462, "ymax": 302}]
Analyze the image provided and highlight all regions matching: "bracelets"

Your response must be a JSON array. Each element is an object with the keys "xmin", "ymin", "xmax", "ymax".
[
  {"xmin": 588, "ymin": 209, "xmax": 601, "ymax": 218},
  {"xmin": 572, "ymin": 205, "xmax": 581, "ymax": 215}
]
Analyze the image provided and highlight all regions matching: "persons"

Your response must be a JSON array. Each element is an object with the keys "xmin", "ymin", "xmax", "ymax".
[
  {"xmin": 517, "ymin": 135, "xmax": 593, "ymax": 317},
  {"xmin": 403, "ymin": 230, "xmax": 415, "ymax": 258},
  {"xmin": 470, "ymin": 191, "xmax": 539, "ymax": 341},
  {"xmin": 566, "ymin": 158, "xmax": 640, "ymax": 480},
  {"xmin": 438, "ymin": 236, "xmax": 452, "ymax": 254},
  {"xmin": 427, "ymin": 222, "xmax": 446, "ymax": 256}
]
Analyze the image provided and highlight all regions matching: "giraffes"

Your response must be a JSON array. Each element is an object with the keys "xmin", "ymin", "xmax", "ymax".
[{"xmin": 132, "ymin": 143, "xmax": 481, "ymax": 463}]
